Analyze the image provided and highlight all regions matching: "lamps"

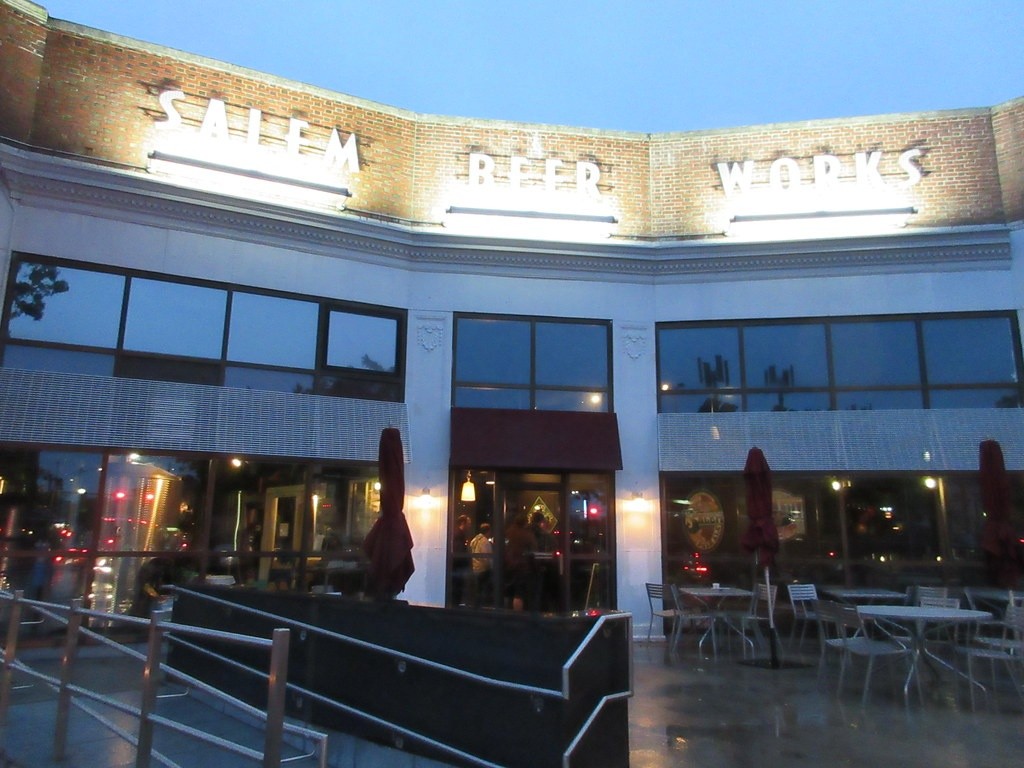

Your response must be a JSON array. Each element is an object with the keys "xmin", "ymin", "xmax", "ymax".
[{"xmin": 91, "ymin": 397, "xmax": 937, "ymax": 574}]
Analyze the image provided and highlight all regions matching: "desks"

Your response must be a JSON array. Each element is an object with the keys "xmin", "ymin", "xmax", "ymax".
[
  {"xmin": 822, "ymin": 585, "xmax": 908, "ymax": 657},
  {"xmin": 678, "ymin": 585, "xmax": 762, "ymax": 657},
  {"xmin": 855, "ymin": 604, "xmax": 992, "ymax": 708}
]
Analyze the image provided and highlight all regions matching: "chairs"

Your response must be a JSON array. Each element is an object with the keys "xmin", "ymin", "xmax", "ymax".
[
  {"xmin": 787, "ymin": 584, "xmax": 830, "ymax": 648},
  {"xmin": 968, "ymin": 604, "xmax": 1024, "ymax": 713},
  {"xmin": 915, "ymin": 587, "xmax": 950, "ymax": 651},
  {"xmin": 646, "ymin": 581, "xmax": 778, "ymax": 659},
  {"xmin": 918, "ymin": 597, "xmax": 961, "ymax": 688},
  {"xmin": 963, "ymin": 587, "xmax": 1021, "ymax": 659},
  {"xmin": 811, "ymin": 598, "xmax": 870, "ymax": 694},
  {"xmin": 1008, "ymin": 591, "xmax": 1023, "ymax": 655},
  {"xmin": 835, "ymin": 601, "xmax": 910, "ymax": 704}
]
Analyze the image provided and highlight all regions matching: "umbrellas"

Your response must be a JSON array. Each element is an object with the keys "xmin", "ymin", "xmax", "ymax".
[
  {"xmin": 978, "ymin": 440, "xmax": 1024, "ymax": 640},
  {"xmin": 361, "ymin": 429, "xmax": 414, "ymax": 599},
  {"xmin": 741, "ymin": 446, "xmax": 780, "ymax": 667}
]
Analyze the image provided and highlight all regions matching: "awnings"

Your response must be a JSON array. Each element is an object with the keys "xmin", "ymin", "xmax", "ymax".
[{"xmin": 452, "ymin": 409, "xmax": 624, "ymax": 473}]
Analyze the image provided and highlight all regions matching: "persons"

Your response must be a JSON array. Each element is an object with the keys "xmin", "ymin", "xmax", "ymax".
[
  {"xmin": 526, "ymin": 510, "xmax": 546, "ymax": 554},
  {"xmin": 469, "ymin": 523, "xmax": 493, "ymax": 607},
  {"xmin": 452, "ymin": 515, "xmax": 473, "ymax": 608},
  {"xmin": 504, "ymin": 513, "xmax": 538, "ymax": 612}
]
